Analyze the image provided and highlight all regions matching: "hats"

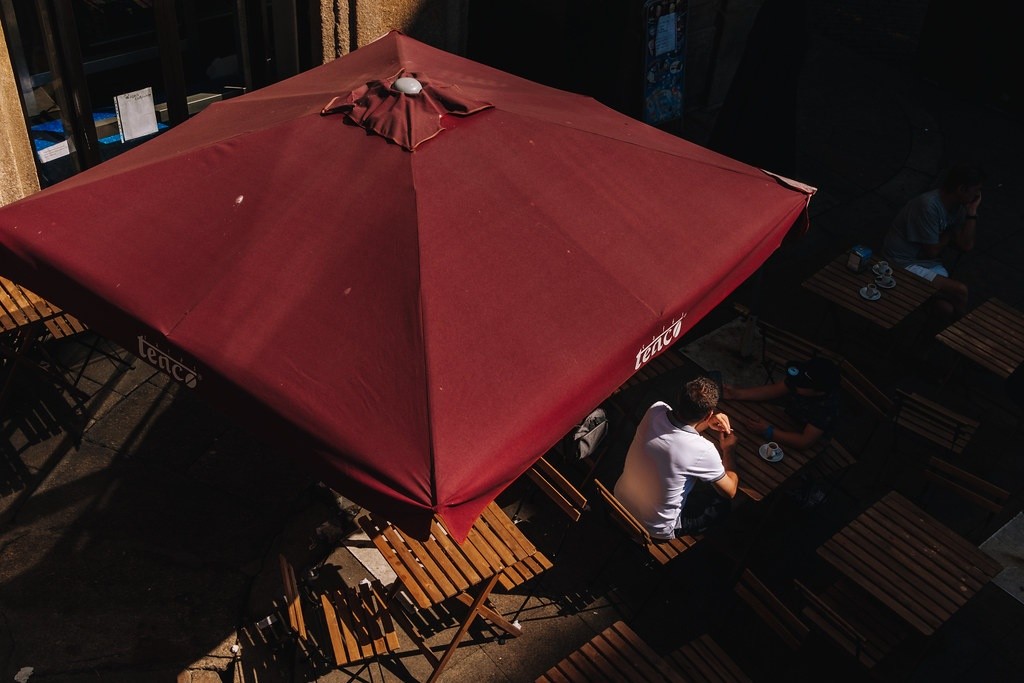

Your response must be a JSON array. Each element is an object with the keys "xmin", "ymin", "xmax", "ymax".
[{"xmin": 785, "ymin": 356, "xmax": 835, "ymax": 389}]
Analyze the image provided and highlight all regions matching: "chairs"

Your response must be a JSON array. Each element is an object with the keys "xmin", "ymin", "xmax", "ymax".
[{"xmin": 244, "ymin": 281, "xmax": 1024, "ymax": 683}]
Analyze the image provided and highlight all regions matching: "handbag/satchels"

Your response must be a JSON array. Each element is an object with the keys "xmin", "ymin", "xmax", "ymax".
[{"xmin": 561, "ymin": 406, "xmax": 610, "ymax": 463}]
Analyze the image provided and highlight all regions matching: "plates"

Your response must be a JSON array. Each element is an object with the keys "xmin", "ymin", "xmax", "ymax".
[
  {"xmin": 872, "ymin": 264, "xmax": 893, "ymax": 276},
  {"xmin": 759, "ymin": 444, "xmax": 783, "ymax": 462},
  {"xmin": 859, "ymin": 287, "xmax": 881, "ymax": 300},
  {"xmin": 875, "ymin": 276, "xmax": 896, "ymax": 288}
]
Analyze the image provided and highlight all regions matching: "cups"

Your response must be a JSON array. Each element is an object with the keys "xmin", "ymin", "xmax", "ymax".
[
  {"xmin": 867, "ymin": 284, "xmax": 877, "ymax": 296},
  {"xmin": 879, "ymin": 261, "xmax": 888, "ymax": 273},
  {"xmin": 882, "ymin": 273, "xmax": 890, "ymax": 285},
  {"xmin": 766, "ymin": 441, "xmax": 778, "ymax": 457}
]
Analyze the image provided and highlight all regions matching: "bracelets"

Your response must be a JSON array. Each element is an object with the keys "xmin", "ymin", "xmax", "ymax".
[
  {"xmin": 763, "ymin": 423, "xmax": 776, "ymax": 442},
  {"xmin": 966, "ymin": 215, "xmax": 979, "ymax": 221}
]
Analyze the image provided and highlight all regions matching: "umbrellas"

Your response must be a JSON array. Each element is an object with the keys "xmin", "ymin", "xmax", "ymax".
[{"xmin": 0, "ymin": 25, "xmax": 817, "ymax": 548}]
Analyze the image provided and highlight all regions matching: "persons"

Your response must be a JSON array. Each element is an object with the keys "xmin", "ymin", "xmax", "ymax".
[
  {"xmin": 722, "ymin": 355, "xmax": 843, "ymax": 453},
  {"xmin": 881, "ymin": 171, "xmax": 985, "ymax": 326},
  {"xmin": 613, "ymin": 377, "xmax": 739, "ymax": 547}
]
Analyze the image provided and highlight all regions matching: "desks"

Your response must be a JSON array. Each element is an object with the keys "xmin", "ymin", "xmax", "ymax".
[
  {"xmin": 799, "ymin": 251, "xmax": 944, "ymax": 361},
  {"xmin": 358, "ymin": 501, "xmax": 537, "ymax": 683},
  {"xmin": 701, "ymin": 399, "xmax": 829, "ymax": 501},
  {"xmin": 815, "ymin": 490, "xmax": 1005, "ymax": 639},
  {"xmin": 533, "ymin": 621, "xmax": 690, "ymax": 683},
  {"xmin": 0, "ymin": 276, "xmax": 137, "ymax": 412},
  {"xmin": 934, "ymin": 297, "xmax": 1024, "ymax": 380}
]
